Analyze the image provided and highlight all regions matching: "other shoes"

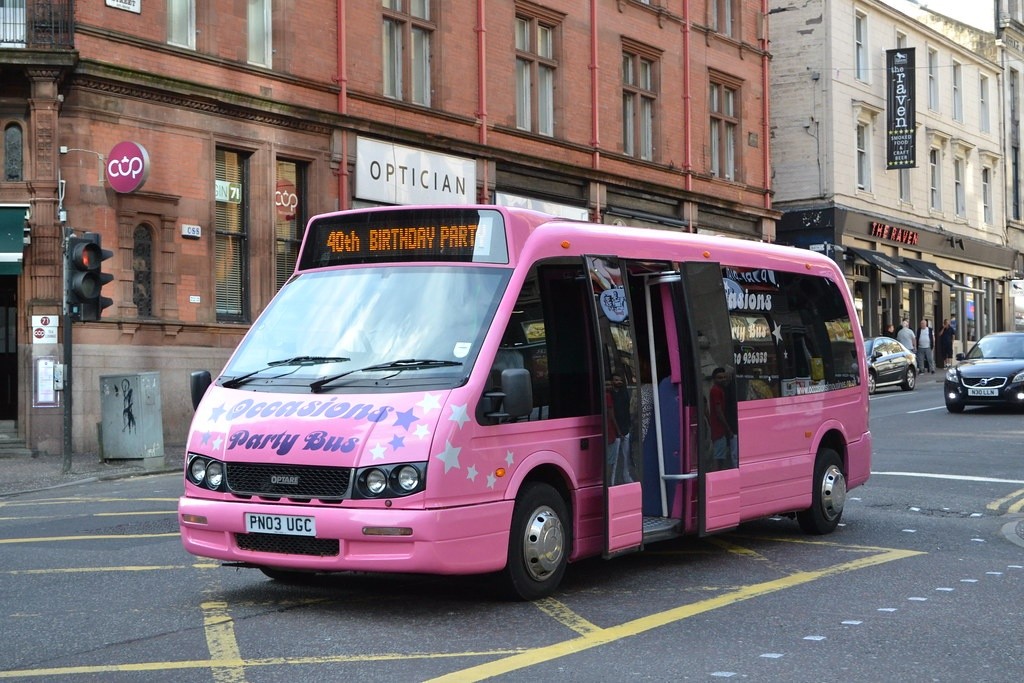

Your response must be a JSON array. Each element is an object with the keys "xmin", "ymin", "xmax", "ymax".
[
  {"xmin": 944, "ymin": 365, "xmax": 952, "ymax": 370},
  {"xmin": 928, "ymin": 370, "xmax": 934, "ymax": 374},
  {"xmin": 918, "ymin": 372, "xmax": 924, "ymax": 375}
]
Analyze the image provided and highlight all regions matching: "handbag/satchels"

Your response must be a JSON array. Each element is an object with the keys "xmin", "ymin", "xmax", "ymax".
[
  {"xmin": 704, "ymin": 416, "xmax": 712, "ymax": 459},
  {"xmin": 730, "ymin": 435, "xmax": 738, "ymax": 468}
]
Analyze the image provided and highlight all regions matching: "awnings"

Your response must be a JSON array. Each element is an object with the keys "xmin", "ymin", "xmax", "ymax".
[
  {"xmin": 900, "ymin": 256, "xmax": 986, "ymax": 295},
  {"xmin": 848, "ymin": 246, "xmax": 936, "ymax": 285}
]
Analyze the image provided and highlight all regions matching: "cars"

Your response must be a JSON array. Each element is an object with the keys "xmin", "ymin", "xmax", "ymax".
[
  {"xmin": 943, "ymin": 332, "xmax": 1024, "ymax": 413},
  {"xmin": 864, "ymin": 336, "xmax": 919, "ymax": 394}
]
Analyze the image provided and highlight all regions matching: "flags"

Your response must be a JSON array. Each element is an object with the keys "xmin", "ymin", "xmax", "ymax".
[{"xmin": 887, "ymin": 49, "xmax": 916, "ymax": 168}]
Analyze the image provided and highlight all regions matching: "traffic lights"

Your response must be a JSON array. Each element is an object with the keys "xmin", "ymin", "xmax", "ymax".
[{"xmin": 68, "ymin": 233, "xmax": 114, "ymax": 322}]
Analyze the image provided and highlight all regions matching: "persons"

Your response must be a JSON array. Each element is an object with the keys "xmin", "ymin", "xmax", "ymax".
[
  {"xmin": 604, "ymin": 375, "xmax": 634, "ymax": 485},
  {"xmin": 710, "ymin": 367, "xmax": 738, "ymax": 469},
  {"xmin": 938, "ymin": 319, "xmax": 955, "ymax": 368},
  {"xmin": 886, "ymin": 318, "xmax": 935, "ymax": 375}
]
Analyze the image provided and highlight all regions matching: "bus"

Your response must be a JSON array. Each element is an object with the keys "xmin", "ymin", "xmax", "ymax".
[{"xmin": 177, "ymin": 203, "xmax": 872, "ymax": 602}]
